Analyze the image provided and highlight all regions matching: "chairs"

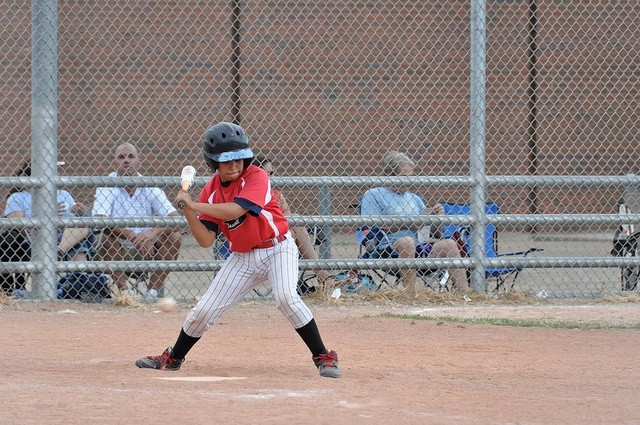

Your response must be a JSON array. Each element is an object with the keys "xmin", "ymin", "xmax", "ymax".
[
  {"xmin": 213, "ymin": 224, "xmax": 319, "ymax": 297},
  {"xmin": 350, "ymin": 197, "xmax": 438, "ymax": 289},
  {"xmin": 87, "ymin": 204, "xmax": 158, "ymax": 297},
  {"xmin": 438, "ymin": 200, "xmax": 542, "ymax": 292},
  {"xmin": 612, "ymin": 187, "xmax": 640, "ymax": 290}
]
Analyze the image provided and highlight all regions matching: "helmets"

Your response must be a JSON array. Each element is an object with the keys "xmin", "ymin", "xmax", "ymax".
[{"xmin": 202, "ymin": 122, "xmax": 254, "ymax": 173}]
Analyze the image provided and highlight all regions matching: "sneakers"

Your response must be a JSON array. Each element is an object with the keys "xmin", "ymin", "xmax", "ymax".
[
  {"xmin": 136, "ymin": 346, "xmax": 186, "ymax": 371},
  {"xmin": 312, "ymin": 348, "xmax": 341, "ymax": 378}
]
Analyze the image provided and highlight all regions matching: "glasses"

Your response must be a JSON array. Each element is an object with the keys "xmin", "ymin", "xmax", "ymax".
[{"xmin": 266, "ymin": 170, "xmax": 274, "ymax": 175}]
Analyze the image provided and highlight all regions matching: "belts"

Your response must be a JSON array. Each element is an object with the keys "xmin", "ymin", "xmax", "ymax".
[{"xmin": 252, "ymin": 235, "xmax": 286, "ymax": 249}]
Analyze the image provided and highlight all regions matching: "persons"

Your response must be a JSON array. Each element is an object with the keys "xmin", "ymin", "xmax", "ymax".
[
  {"xmin": 91, "ymin": 142, "xmax": 181, "ymax": 296},
  {"xmin": 135, "ymin": 119, "xmax": 341, "ymax": 379},
  {"xmin": 360, "ymin": 150, "xmax": 468, "ymax": 295},
  {"xmin": 249, "ymin": 156, "xmax": 355, "ymax": 290},
  {"xmin": 1, "ymin": 157, "xmax": 92, "ymax": 296}
]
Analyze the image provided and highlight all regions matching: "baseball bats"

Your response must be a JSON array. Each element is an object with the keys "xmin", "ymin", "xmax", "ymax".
[{"xmin": 177, "ymin": 165, "xmax": 196, "ymax": 209}]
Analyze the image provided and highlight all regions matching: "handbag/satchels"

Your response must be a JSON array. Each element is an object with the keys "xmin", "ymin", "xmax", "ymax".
[{"xmin": 57, "ymin": 250, "xmax": 112, "ymax": 299}]
